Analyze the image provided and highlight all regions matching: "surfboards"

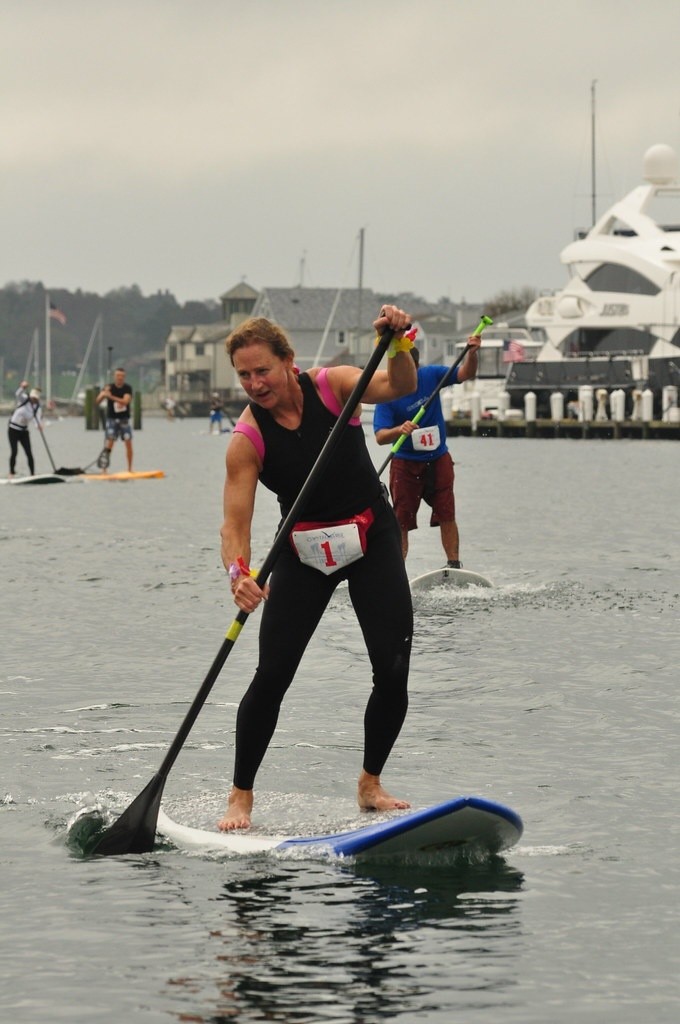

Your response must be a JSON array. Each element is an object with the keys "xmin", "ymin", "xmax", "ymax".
[
  {"xmin": 0, "ymin": 473, "xmax": 66, "ymax": 485},
  {"xmin": 119, "ymin": 796, "xmax": 524, "ymax": 861},
  {"xmin": 77, "ymin": 469, "xmax": 163, "ymax": 482},
  {"xmin": 409, "ymin": 567, "xmax": 495, "ymax": 594}
]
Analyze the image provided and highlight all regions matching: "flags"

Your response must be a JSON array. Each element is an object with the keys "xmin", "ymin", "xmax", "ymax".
[{"xmin": 502, "ymin": 338, "xmax": 526, "ymax": 362}]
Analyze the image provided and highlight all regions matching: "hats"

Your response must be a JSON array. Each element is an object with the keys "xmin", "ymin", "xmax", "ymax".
[{"xmin": 30, "ymin": 389, "xmax": 41, "ymax": 399}]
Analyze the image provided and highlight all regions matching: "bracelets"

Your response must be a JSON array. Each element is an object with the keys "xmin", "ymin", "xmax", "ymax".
[
  {"xmin": 229, "ymin": 557, "xmax": 258, "ymax": 594},
  {"xmin": 375, "ymin": 327, "xmax": 418, "ymax": 358}
]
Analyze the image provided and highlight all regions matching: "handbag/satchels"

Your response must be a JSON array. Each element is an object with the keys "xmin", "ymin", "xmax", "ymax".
[{"xmin": 278, "ymin": 482, "xmax": 389, "ymax": 577}]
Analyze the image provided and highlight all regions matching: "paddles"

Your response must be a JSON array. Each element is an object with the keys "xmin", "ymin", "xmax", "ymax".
[
  {"xmin": 377, "ymin": 315, "xmax": 493, "ymax": 477},
  {"xmin": 24, "ymin": 385, "xmax": 56, "ymax": 473},
  {"xmin": 214, "ymin": 398, "xmax": 237, "ymax": 427},
  {"xmin": 84, "ymin": 312, "xmax": 413, "ymax": 856}
]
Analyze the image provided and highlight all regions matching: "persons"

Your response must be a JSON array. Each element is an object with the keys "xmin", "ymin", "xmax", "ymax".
[
  {"xmin": 218, "ymin": 303, "xmax": 418, "ymax": 830},
  {"xmin": 483, "ymin": 407, "xmax": 491, "ymax": 417},
  {"xmin": 629, "ymin": 387, "xmax": 642, "ymax": 421},
  {"xmin": 166, "ymin": 395, "xmax": 175, "ymax": 419},
  {"xmin": 208, "ymin": 392, "xmax": 224, "ymax": 434},
  {"xmin": 595, "ymin": 389, "xmax": 609, "ymax": 421},
  {"xmin": 6, "ymin": 380, "xmax": 43, "ymax": 478},
  {"xmin": 95, "ymin": 367, "xmax": 133, "ymax": 474},
  {"xmin": 372, "ymin": 334, "xmax": 482, "ymax": 570}
]
